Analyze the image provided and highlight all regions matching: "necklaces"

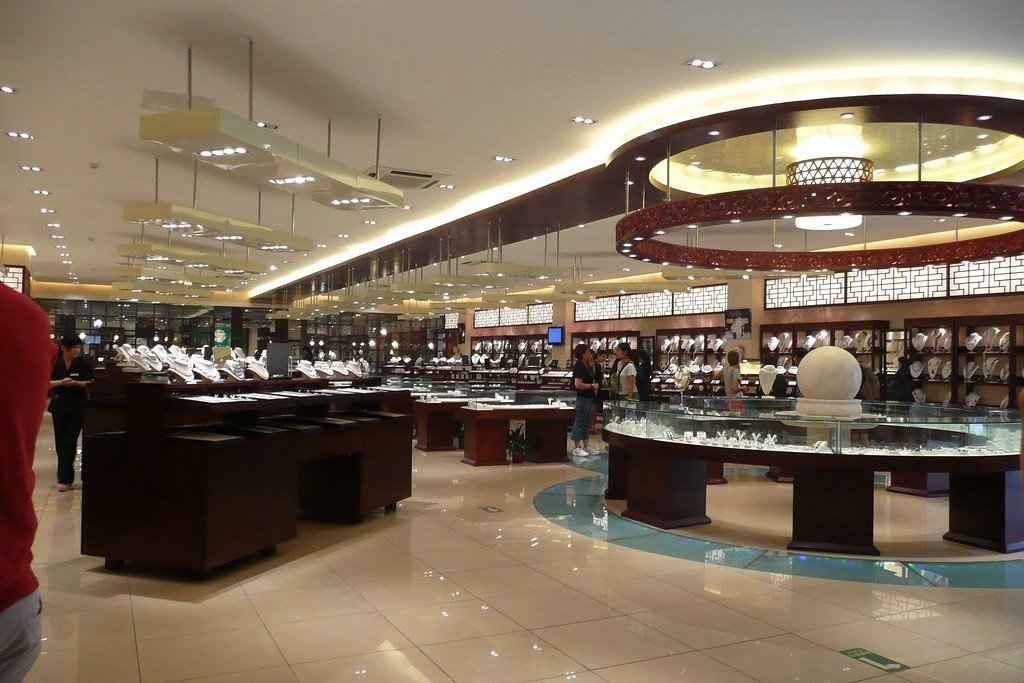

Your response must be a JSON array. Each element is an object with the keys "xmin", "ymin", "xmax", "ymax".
[
  {"xmin": 591, "ymin": 330, "xmax": 876, "ymax": 392},
  {"xmin": 121, "ymin": 343, "xmax": 363, "ymax": 381},
  {"xmin": 908, "ymin": 327, "xmax": 1009, "ymax": 409}
]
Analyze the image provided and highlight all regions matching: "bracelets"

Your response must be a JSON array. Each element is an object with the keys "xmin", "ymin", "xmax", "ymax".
[{"xmin": 591, "ymin": 384, "xmax": 594, "ymax": 389}]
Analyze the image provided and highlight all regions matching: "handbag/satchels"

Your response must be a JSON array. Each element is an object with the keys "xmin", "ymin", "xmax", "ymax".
[
  {"xmin": 716, "ymin": 386, "xmax": 726, "ymax": 396},
  {"xmin": 611, "ymin": 393, "xmax": 619, "ymax": 400}
]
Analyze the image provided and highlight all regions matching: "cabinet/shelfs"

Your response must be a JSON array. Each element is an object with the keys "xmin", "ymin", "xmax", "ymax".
[
  {"xmin": 470, "ymin": 313, "xmax": 1024, "ymax": 419},
  {"xmin": 299, "ymin": 320, "xmax": 450, "ymax": 364}
]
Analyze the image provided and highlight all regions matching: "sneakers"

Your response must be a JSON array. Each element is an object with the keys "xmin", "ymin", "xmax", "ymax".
[
  {"xmin": 572, "ymin": 456, "xmax": 588, "ymax": 465},
  {"xmin": 572, "ymin": 446, "xmax": 589, "ymax": 457},
  {"xmin": 582, "ymin": 446, "xmax": 600, "ymax": 455},
  {"xmin": 588, "ymin": 456, "xmax": 602, "ymax": 465}
]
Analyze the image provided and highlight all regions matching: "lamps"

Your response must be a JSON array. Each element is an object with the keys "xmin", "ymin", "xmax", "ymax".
[{"xmin": 113, "ymin": 36, "xmax": 1023, "ymax": 300}]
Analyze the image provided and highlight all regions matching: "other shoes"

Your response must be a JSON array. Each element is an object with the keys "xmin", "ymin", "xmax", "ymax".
[
  {"xmin": 54, "ymin": 483, "xmax": 74, "ymax": 492},
  {"xmin": 605, "ymin": 446, "xmax": 609, "ymax": 451},
  {"xmin": 590, "ymin": 429, "xmax": 600, "ymax": 435}
]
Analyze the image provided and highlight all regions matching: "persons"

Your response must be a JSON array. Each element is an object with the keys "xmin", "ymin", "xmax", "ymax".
[
  {"xmin": 879, "ymin": 357, "xmax": 910, "ymax": 402},
  {"xmin": 586, "ymin": 348, "xmax": 652, "ymax": 452},
  {"xmin": 569, "ymin": 455, "xmax": 606, "ymax": 568},
  {"xmin": 0, "ymin": 281, "xmax": 57, "ymax": 683},
  {"xmin": 570, "ymin": 344, "xmax": 601, "ymax": 457},
  {"xmin": 756, "ymin": 352, "xmax": 786, "ymax": 397},
  {"xmin": 723, "ymin": 350, "xmax": 750, "ymax": 410},
  {"xmin": 792, "ymin": 346, "xmax": 877, "ymax": 400},
  {"xmin": 605, "ymin": 342, "xmax": 640, "ymax": 450},
  {"xmin": 46, "ymin": 333, "xmax": 87, "ymax": 492}
]
